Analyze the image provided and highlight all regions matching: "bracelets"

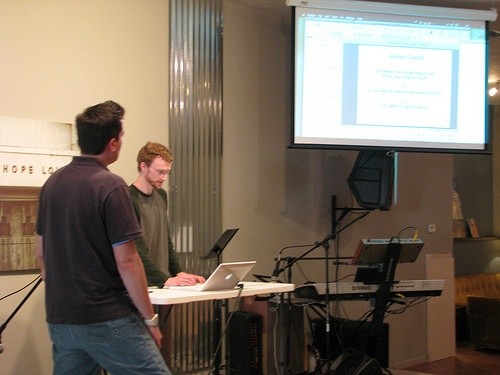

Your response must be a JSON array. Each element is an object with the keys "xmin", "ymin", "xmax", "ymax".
[{"xmin": 145, "ymin": 314, "xmax": 159, "ymax": 326}]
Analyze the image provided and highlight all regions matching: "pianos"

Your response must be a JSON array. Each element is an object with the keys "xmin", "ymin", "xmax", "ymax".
[{"xmin": 292, "ymin": 237, "xmax": 446, "ymax": 362}]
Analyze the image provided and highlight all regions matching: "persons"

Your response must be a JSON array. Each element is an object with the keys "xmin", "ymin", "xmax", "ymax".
[
  {"xmin": 128, "ymin": 141, "xmax": 206, "ymax": 369},
  {"xmin": 35, "ymin": 100, "xmax": 172, "ymax": 375}
]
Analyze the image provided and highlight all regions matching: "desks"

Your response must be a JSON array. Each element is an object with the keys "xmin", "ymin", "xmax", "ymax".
[{"xmin": 148, "ymin": 281, "xmax": 295, "ymax": 375}]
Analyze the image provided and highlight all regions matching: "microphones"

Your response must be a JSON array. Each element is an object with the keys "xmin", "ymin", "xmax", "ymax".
[{"xmin": 272, "ymin": 252, "xmax": 282, "ymax": 283}]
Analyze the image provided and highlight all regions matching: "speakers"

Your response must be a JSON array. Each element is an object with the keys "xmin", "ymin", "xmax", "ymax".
[
  {"xmin": 323, "ymin": 347, "xmax": 383, "ymax": 375},
  {"xmin": 348, "ymin": 150, "xmax": 392, "ymax": 210},
  {"xmin": 228, "ymin": 311, "xmax": 263, "ymax": 375}
]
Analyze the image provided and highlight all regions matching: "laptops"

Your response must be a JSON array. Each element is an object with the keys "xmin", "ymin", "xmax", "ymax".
[{"xmin": 169, "ymin": 261, "xmax": 257, "ymax": 292}]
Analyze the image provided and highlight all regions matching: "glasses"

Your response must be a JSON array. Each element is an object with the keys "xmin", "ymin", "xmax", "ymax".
[{"xmin": 148, "ymin": 167, "xmax": 172, "ymax": 177}]
